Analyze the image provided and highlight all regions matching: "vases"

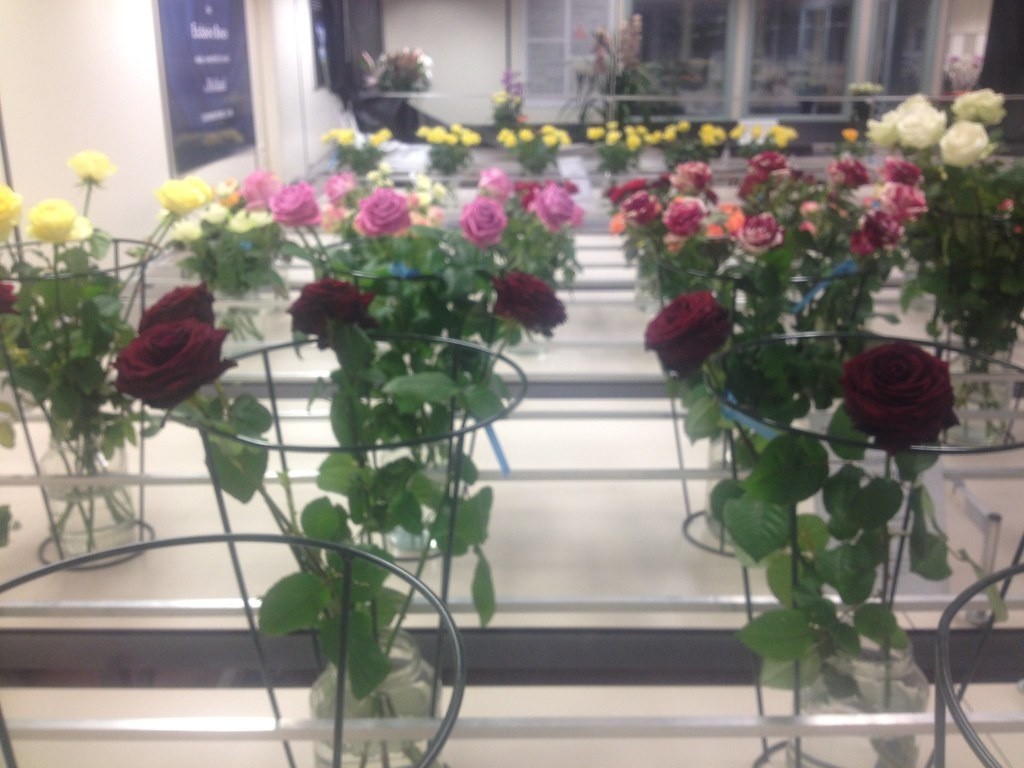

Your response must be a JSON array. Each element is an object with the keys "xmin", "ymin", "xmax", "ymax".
[
  {"xmin": 785, "ymin": 640, "xmax": 929, "ymax": 768},
  {"xmin": 310, "ymin": 627, "xmax": 444, "ymax": 768},
  {"xmin": 705, "ymin": 427, "xmax": 751, "ymax": 543},
  {"xmin": 944, "ymin": 345, "xmax": 1013, "ymax": 447},
  {"xmin": 38, "ymin": 438, "xmax": 135, "ymax": 556},
  {"xmin": 385, "ymin": 469, "xmax": 450, "ymax": 551}
]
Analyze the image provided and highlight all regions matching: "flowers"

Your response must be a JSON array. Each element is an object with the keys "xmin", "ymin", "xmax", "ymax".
[{"xmin": 0, "ymin": 13, "xmax": 1024, "ymax": 768}]
